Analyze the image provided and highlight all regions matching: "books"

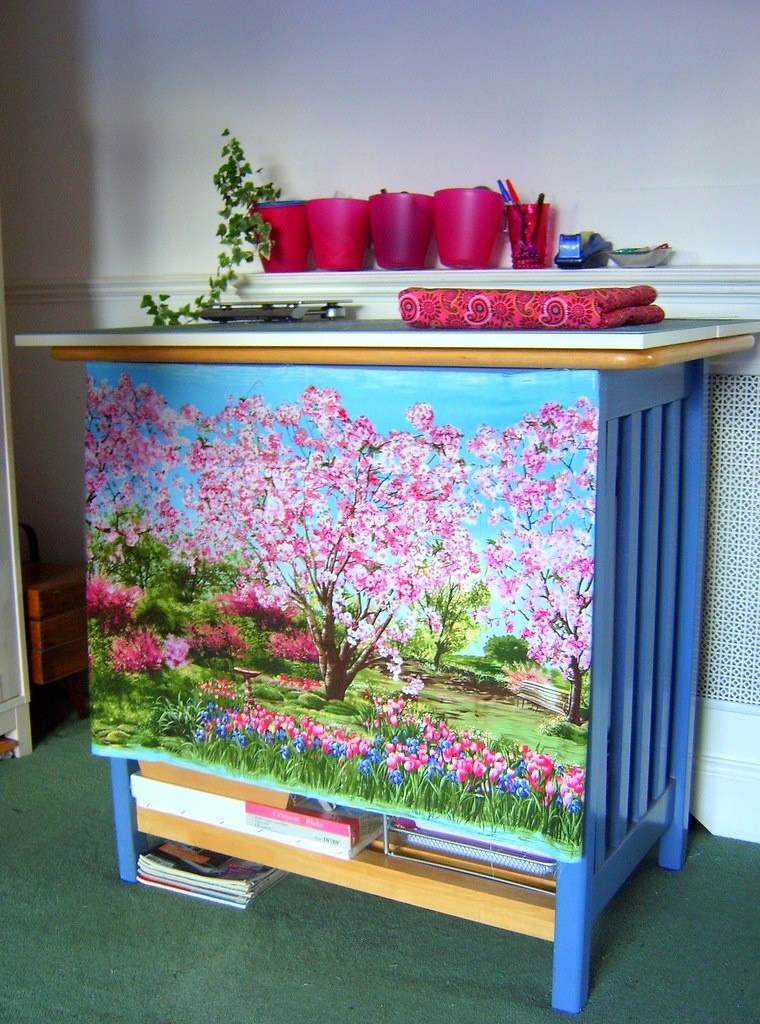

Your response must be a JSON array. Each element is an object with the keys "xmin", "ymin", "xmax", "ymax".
[{"xmin": 136, "ymin": 840, "xmax": 289, "ymax": 910}]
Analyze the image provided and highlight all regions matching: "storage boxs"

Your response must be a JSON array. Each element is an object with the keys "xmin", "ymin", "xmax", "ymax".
[{"xmin": 20, "ymin": 560, "xmax": 89, "ymax": 686}]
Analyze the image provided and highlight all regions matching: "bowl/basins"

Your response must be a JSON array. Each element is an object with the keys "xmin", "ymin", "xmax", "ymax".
[{"xmin": 605, "ymin": 248, "xmax": 672, "ymax": 268}]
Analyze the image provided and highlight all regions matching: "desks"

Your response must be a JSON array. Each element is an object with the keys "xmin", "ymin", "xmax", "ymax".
[{"xmin": 10, "ymin": 313, "xmax": 760, "ymax": 1014}]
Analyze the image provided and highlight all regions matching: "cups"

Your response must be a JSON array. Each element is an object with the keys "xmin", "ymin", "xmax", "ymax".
[
  {"xmin": 250, "ymin": 200, "xmax": 311, "ymax": 273},
  {"xmin": 368, "ymin": 193, "xmax": 435, "ymax": 270},
  {"xmin": 305, "ymin": 198, "xmax": 370, "ymax": 272},
  {"xmin": 434, "ymin": 188, "xmax": 505, "ymax": 269},
  {"xmin": 505, "ymin": 203, "xmax": 551, "ymax": 270}
]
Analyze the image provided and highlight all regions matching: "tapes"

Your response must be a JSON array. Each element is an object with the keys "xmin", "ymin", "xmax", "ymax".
[{"xmin": 579, "ymin": 231, "xmax": 594, "ymax": 245}]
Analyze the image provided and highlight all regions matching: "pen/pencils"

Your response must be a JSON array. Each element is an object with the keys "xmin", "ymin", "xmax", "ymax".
[{"xmin": 497, "ymin": 179, "xmax": 545, "ymax": 264}]
